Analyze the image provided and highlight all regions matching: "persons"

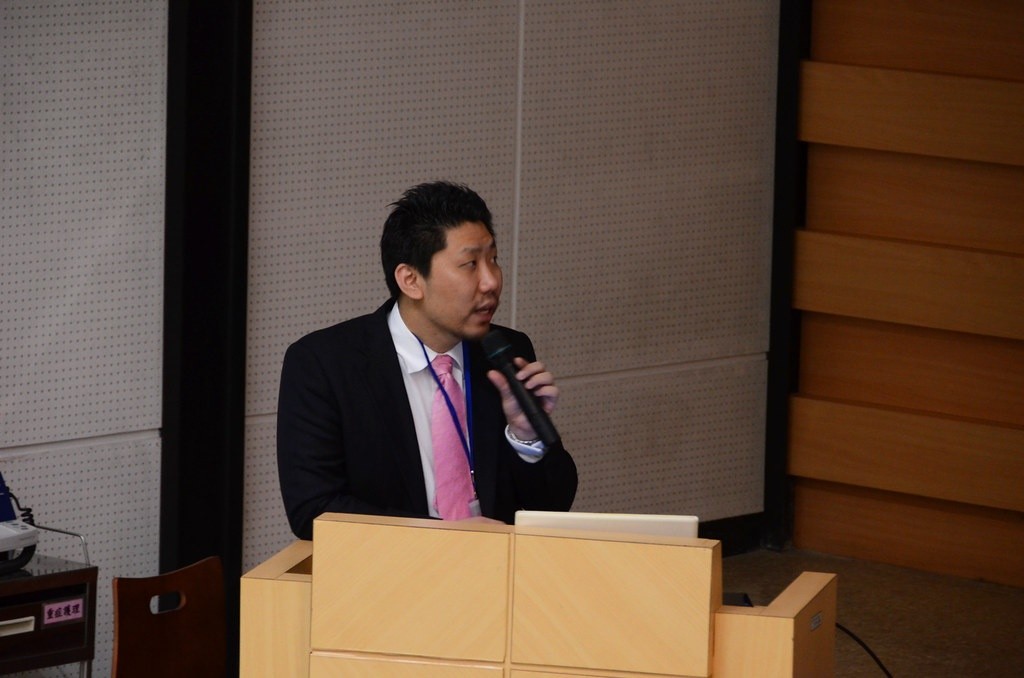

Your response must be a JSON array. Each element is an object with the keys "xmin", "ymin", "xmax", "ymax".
[{"xmin": 275, "ymin": 181, "xmax": 578, "ymax": 541}]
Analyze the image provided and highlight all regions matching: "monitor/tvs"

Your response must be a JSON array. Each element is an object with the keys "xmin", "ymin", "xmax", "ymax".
[{"xmin": 515, "ymin": 510, "xmax": 699, "ymax": 538}]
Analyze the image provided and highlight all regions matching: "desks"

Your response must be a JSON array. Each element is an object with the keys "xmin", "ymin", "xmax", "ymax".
[{"xmin": 0, "ymin": 551, "xmax": 99, "ymax": 678}]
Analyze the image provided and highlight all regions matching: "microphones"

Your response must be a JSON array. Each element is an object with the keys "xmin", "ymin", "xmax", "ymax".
[{"xmin": 480, "ymin": 328, "xmax": 561, "ymax": 448}]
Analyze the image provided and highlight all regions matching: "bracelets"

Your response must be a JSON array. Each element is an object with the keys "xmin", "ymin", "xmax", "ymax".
[{"xmin": 510, "ymin": 433, "xmax": 539, "ymax": 445}]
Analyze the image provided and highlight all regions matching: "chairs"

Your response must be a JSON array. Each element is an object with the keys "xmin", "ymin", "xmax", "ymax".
[{"xmin": 111, "ymin": 555, "xmax": 227, "ymax": 678}]
